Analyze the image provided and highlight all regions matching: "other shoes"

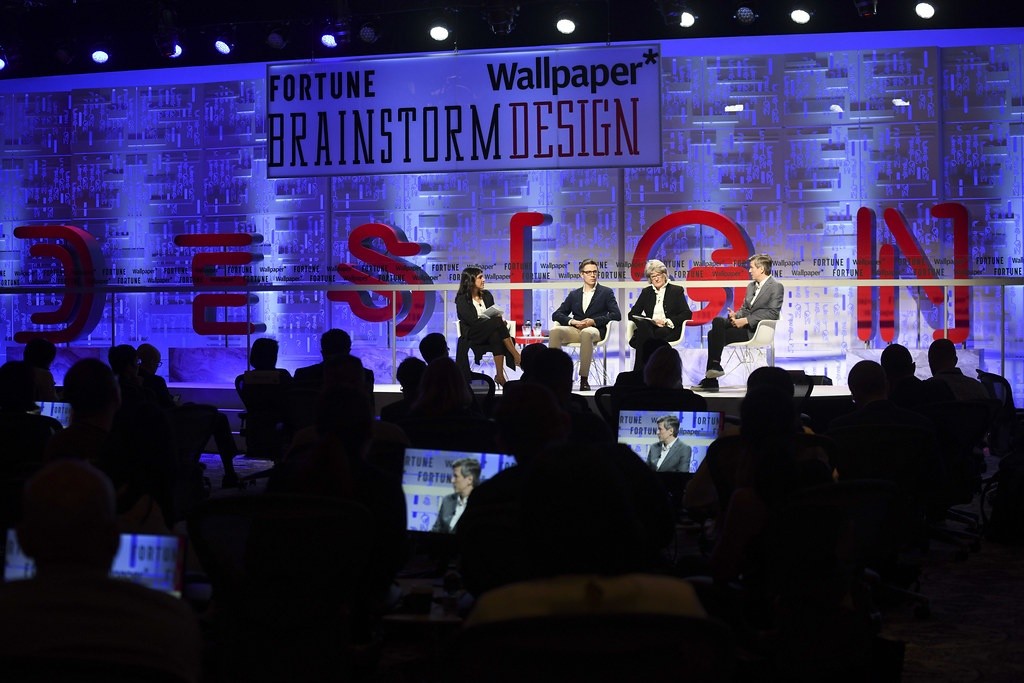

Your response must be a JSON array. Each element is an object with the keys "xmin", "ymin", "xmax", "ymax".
[{"xmin": 580, "ymin": 380, "xmax": 590, "ymax": 390}]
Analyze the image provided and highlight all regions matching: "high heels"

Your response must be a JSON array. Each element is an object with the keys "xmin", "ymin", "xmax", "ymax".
[{"xmin": 494, "ymin": 375, "xmax": 506, "ymax": 390}]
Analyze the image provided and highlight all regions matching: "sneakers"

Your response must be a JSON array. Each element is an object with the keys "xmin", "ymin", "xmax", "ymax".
[
  {"xmin": 706, "ymin": 363, "xmax": 725, "ymax": 378},
  {"xmin": 691, "ymin": 379, "xmax": 719, "ymax": 390}
]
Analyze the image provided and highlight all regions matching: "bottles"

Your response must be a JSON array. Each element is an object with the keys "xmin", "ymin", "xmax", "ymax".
[
  {"xmin": 535, "ymin": 320, "xmax": 542, "ymax": 328},
  {"xmin": 524, "ymin": 320, "xmax": 532, "ymax": 331}
]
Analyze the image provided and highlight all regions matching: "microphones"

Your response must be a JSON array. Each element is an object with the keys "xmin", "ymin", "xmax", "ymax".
[
  {"xmin": 659, "ymin": 454, "xmax": 661, "ymax": 458},
  {"xmin": 753, "ymin": 293, "xmax": 755, "ymax": 295},
  {"xmin": 479, "ymin": 303, "xmax": 481, "ymax": 306},
  {"xmin": 453, "ymin": 511, "xmax": 456, "ymax": 515},
  {"xmin": 658, "ymin": 300, "xmax": 660, "ymax": 302}
]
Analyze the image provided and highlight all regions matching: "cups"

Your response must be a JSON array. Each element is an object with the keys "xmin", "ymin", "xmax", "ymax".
[
  {"xmin": 521, "ymin": 325, "xmax": 531, "ymax": 337},
  {"xmin": 533, "ymin": 326, "xmax": 542, "ymax": 337}
]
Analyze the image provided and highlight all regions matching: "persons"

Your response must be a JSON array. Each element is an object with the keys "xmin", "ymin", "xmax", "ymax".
[
  {"xmin": 690, "ymin": 254, "xmax": 784, "ymax": 392},
  {"xmin": 647, "ymin": 416, "xmax": 691, "ymax": 472},
  {"xmin": 628, "ymin": 260, "xmax": 692, "ymax": 371},
  {"xmin": 549, "ymin": 259, "xmax": 622, "ymax": 390},
  {"xmin": 455, "ymin": 267, "xmax": 522, "ymax": 390},
  {"xmin": 432, "ymin": 458, "xmax": 480, "ymax": 532},
  {"xmin": 0, "ymin": 340, "xmax": 1023, "ymax": 683}
]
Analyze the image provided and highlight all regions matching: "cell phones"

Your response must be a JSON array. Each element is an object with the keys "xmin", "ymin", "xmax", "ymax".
[{"xmin": 173, "ymin": 393, "xmax": 182, "ymax": 401}]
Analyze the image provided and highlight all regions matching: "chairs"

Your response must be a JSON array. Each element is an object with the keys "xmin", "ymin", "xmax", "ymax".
[
  {"xmin": 186, "ymin": 493, "xmax": 417, "ymax": 660},
  {"xmin": 595, "ymin": 388, "xmax": 1002, "ymax": 673},
  {"xmin": 717, "ymin": 315, "xmax": 783, "ymax": 385},
  {"xmin": 452, "ymin": 319, "xmax": 516, "ymax": 383},
  {"xmin": 553, "ymin": 320, "xmax": 614, "ymax": 386},
  {"xmin": 235, "ymin": 372, "xmax": 301, "ymax": 437},
  {"xmin": 626, "ymin": 320, "xmax": 694, "ymax": 347}
]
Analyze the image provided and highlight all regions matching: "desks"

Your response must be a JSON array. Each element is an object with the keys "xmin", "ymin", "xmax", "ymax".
[{"xmin": 516, "ymin": 336, "xmax": 550, "ymax": 346}]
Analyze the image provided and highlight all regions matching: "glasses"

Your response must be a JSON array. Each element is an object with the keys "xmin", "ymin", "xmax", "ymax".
[
  {"xmin": 582, "ymin": 270, "xmax": 598, "ymax": 275},
  {"xmin": 650, "ymin": 273, "xmax": 662, "ymax": 280}
]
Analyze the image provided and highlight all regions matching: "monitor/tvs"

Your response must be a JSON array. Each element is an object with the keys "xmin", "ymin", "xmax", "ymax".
[
  {"xmin": 618, "ymin": 410, "xmax": 722, "ymax": 473},
  {"xmin": 4, "ymin": 528, "xmax": 188, "ymax": 600},
  {"xmin": 27, "ymin": 401, "xmax": 72, "ymax": 428},
  {"xmin": 402, "ymin": 448, "xmax": 517, "ymax": 537}
]
